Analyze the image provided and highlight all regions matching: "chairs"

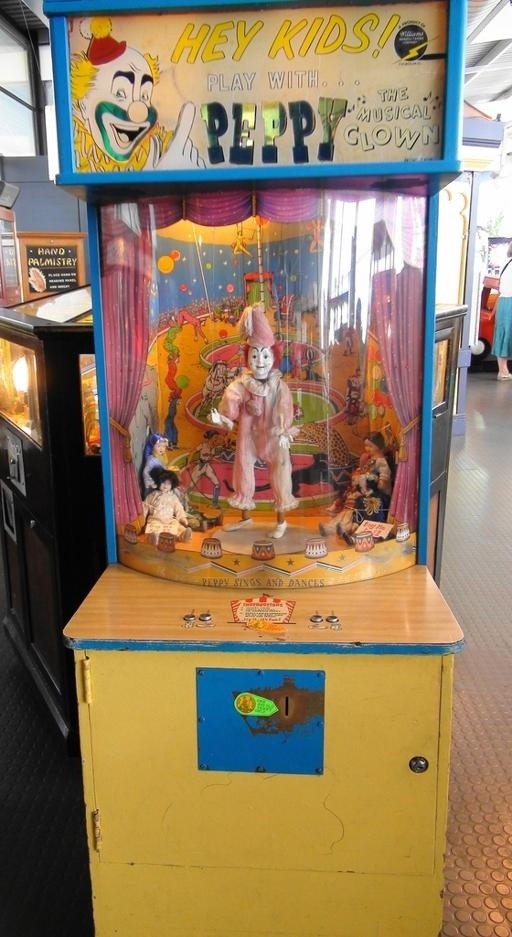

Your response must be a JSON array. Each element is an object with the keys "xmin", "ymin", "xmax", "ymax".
[{"xmin": 498, "ymin": 371, "xmax": 512, "ymax": 380}]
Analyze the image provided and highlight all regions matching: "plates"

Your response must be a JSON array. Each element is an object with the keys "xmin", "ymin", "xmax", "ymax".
[
  {"xmin": 0, "ymin": 286, "xmax": 469, "ymax": 750},
  {"xmin": 41, "ymin": 0, "xmax": 468, "ymax": 937}
]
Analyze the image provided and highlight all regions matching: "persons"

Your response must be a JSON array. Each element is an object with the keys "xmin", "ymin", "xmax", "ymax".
[
  {"xmin": 143, "ymin": 434, "xmax": 168, "ymax": 501},
  {"xmin": 318, "ymin": 431, "xmax": 392, "ymax": 546},
  {"xmin": 490, "ymin": 242, "xmax": 512, "ymax": 381},
  {"xmin": 206, "ymin": 307, "xmax": 300, "ymax": 539},
  {"xmin": 138, "ymin": 466, "xmax": 192, "ymax": 545}
]
